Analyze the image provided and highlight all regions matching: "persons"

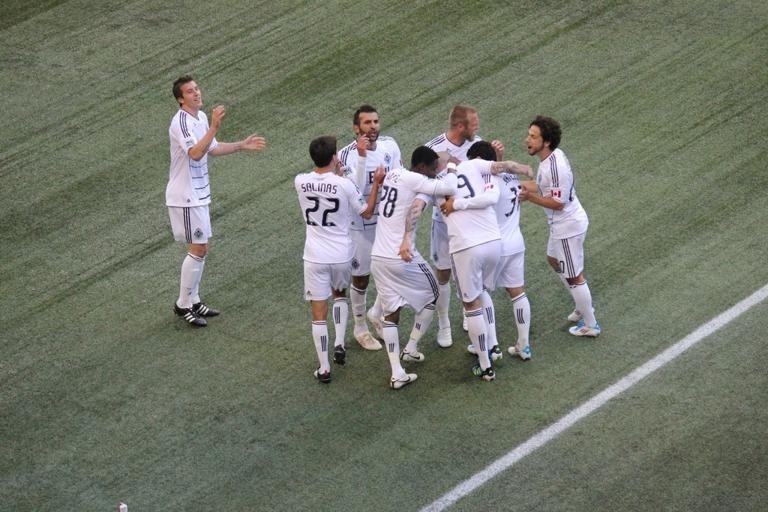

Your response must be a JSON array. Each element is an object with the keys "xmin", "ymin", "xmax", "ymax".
[
  {"xmin": 517, "ymin": 115, "xmax": 602, "ymax": 338},
  {"xmin": 164, "ymin": 74, "xmax": 267, "ymax": 329},
  {"xmin": 293, "ymin": 101, "xmax": 534, "ymax": 390}
]
